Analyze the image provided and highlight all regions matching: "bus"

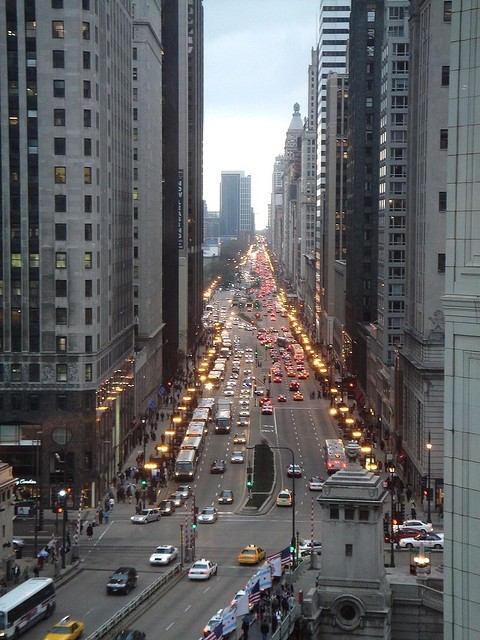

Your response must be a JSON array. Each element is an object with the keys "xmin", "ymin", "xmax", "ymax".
[
  {"xmin": 191, "ymin": 408, "xmax": 211, "ymax": 428},
  {"xmin": 323, "ymin": 438, "xmax": 347, "ymax": 474},
  {"xmin": 172, "ymin": 449, "xmax": 198, "ymax": 482},
  {"xmin": 180, "ymin": 436, "xmax": 203, "ymax": 462},
  {"xmin": 0, "ymin": 576, "xmax": 57, "ymax": 640},
  {"xmin": 185, "ymin": 421, "xmax": 207, "ymax": 444},
  {"xmin": 215, "ymin": 409, "xmax": 231, "ymax": 434},
  {"xmin": 197, "ymin": 398, "xmax": 216, "ymax": 417}
]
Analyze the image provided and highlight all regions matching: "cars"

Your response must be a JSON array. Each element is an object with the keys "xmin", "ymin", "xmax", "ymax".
[
  {"xmin": 235, "ymin": 398, "xmax": 273, "ymax": 428},
  {"xmin": 203, "ymin": 609, "xmax": 224, "ymax": 638},
  {"xmin": 202, "ymin": 255, "xmax": 313, "ymax": 402},
  {"xmin": 197, "ymin": 506, "xmax": 218, "ymax": 524},
  {"xmin": 399, "ymin": 532, "xmax": 444, "ymax": 549},
  {"xmin": 174, "ymin": 485, "xmax": 194, "ymax": 499},
  {"xmin": 285, "ymin": 464, "xmax": 302, "ymax": 478},
  {"xmin": 130, "ymin": 508, "xmax": 162, "ymax": 525},
  {"xmin": 384, "ymin": 528, "xmax": 424, "ymax": 544},
  {"xmin": 232, "ymin": 432, "xmax": 247, "ymax": 445},
  {"xmin": 308, "ymin": 476, "xmax": 325, "ymax": 490},
  {"xmin": 393, "ymin": 519, "xmax": 434, "ymax": 534},
  {"xmin": 230, "ymin": 451, "xmax": 244, "ymax": 464},
  {"xmin": 168, "ymin": 493, "xmax": 186, "ymax": 508},
  {"xmin": 149, "ymin": 545, "xmax": 179, "ymax": 566},
  {"xmin": 230, "ymin": 589, "xmax": 245, "ymax": 605},
  {"xmin": 187, "ymin": 559, "xmax": 218, "ymax": 580},
  {"xmin": 156, "ymin": 500, "xmax": 176, "ymax": 515},
  {"xmin": 217, "ymin": 489, "xmax": 234, "ymax": 505},
  {"xmin": 275, "ymin": 488, "xmax": 293, "ymax": 506},
  {"xmin": 238, "ymin": 544, "xmax": 266, "ymax": 565},
  {"xmin": 113, "ymin": 629, "xmax": 146, "ymax": 640},
  {"xmin": 210, "ymin": 460, "xmax": 227, "ymax": 474},
  {"xmin": 299, "ymin": 540, "xmax": 321, "ymax": 555},
  {"xmin": 41, "ymin": 614, "xmax": 85, "ymax": 640}
]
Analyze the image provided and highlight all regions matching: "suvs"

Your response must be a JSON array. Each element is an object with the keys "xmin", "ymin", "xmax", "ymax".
[{"xmin": 105, "ymin": 566, "xmax": 138, "ymax": 596}]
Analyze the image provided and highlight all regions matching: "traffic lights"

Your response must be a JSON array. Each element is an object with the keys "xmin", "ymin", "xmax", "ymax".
[
  {"xmin": 290, "ymin": 547, "xmax": 294, "ymax": 552},
  {"xmin": 192, "ymin": 524, "xmax": 196, "ymax": 528},
  {"xmin": 392, "ymin": 518, "xmax": 399, "ymax": 525},
  {"xmin": 423, "ymin": 488, "xmax": 429, "ymax": 497},
  {"xmin": 246, "ymin": 482, "xmax": 252, "ymax": 486},
  {"xmin": 141, "ymin": 481, "xmax": 147, "ymax": 485}
]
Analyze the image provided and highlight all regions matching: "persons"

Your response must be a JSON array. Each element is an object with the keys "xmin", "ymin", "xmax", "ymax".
[
  {"xmin": 116, "ymin": 451, "xmax": 146, "ymax": 503},
  {"xmin": 33, "ymin": 564, "xmax": 40, "ymax": 575},
  {"xmin": 160, "ymin": 433, "xmax": 165, "ymax": 445},
  {"xmin": 405, "ymin": 487, "xmax": 412, "ymax": 502},
  {"xmin": 46, "ymin": 533, "xmax": 70, "ymax": 563},
  {"xmin": 10, "ymin": 563, "xmax": 20, "ymax": 584},
  {"xmin": 410, "ymin": 508, "xmax": 417, "ymax": 520},
  {"xmin": 258, "ymin": 583, "xmax": 296, "ymax": 637},
  {"xmin": 96, "ymin": 493, "xmax": 116, "ymax": 525},
  {"xmin": 23, "ymin": 566, "xmax": 30, "ymax": 579},
  {"xmin": 38, "ymin": 555, "xmax": 46, "ymax": 570},
  {"xmin": 86, "ymin": 524, "xmax": 94, "ymax": 541}
]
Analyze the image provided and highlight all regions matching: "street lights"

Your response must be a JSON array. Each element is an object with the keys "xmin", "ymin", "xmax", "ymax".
[
  {"xmin": 387, "ymin": 462, "xmax": 396, "ymax": 567},
  {"xmin": 58, "ymin": 488, "xmax": 68, "ymax": 568},
  {"xmin": 245, "ymin": 445, "xmax": 295, "ymax": 572},
  {"xmin": 426, "ymin": 429, "xmax": 433, "ymax": 523}
]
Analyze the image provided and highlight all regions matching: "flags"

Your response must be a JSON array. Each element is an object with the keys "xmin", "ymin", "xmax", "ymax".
[
  {"xmin": 249, "ymin": 545, "xmax": 291, "ymax": 605},
  {"xmin": 201, "ymin": 589, "xmax": 248, "ymax": 640}
]
